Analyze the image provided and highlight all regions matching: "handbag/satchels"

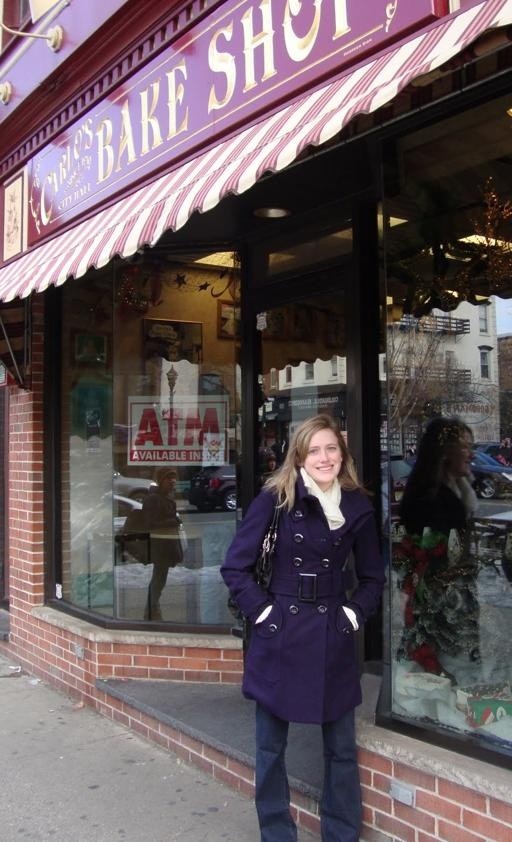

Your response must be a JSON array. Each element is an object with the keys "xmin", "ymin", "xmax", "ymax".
[{"xmin": 227, "ymin": 529, "xmax": 277, "ymax": 628}]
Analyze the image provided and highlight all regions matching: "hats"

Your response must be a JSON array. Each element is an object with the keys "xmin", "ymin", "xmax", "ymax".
[{"xmin": 155, "ymin": 468, "xmax": 176, "ymax": 482}]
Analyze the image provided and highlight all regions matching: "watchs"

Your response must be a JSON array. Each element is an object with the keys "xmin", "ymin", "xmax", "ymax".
[{"xmin": 221, "ymin": 413, "xmax": 389, "ymax": 841}]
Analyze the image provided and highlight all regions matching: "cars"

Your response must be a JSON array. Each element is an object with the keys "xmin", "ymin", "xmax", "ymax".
[
  {"xmin": 112, "ymin": 466, "xmax": 236, "ymax": 564},
  {"xmin": 390, "ymin": 442, "xmax": 512, "ymax": 535}
]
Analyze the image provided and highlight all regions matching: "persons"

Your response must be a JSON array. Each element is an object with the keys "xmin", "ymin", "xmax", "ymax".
[
  {"xmin": 138, "ymin": 466, "xmax": 184, "ymax": 622},
  {"xmin": 399, "ymin": 416, "xmax": 485, "ymax": 687},
  {"xmin": 255, "ymin": 445, "xmax": 280, "ymax": 482},
  {"xmin": 498, "ymin": 436, "xmax": 512, "ymax": 466},
  {"xmin": 407, "ymin": 443, "xmax": 416, "ymax": 457}
]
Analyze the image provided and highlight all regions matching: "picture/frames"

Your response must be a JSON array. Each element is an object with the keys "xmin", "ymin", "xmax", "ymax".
[
  {"xmin": 215, "ymin": 298, "xmax": 349, "ymax": 349},
  {"xmin": 142, "ymin": 317, "xmax": 203, "ymax": 390},
  {"xmin": 69, "ymin": 326, "xmax": 114, "ymax": 370}
]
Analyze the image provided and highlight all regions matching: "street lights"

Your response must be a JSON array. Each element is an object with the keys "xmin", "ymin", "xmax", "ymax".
[{"xmin": 166, "ymin": 364, "xmax": 179, "ymax": 419}]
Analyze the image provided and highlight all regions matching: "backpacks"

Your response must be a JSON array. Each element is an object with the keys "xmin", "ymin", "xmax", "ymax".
[{"xmin": 115, "ymin": 509, "xmax": 150, "ymax": 567}]
